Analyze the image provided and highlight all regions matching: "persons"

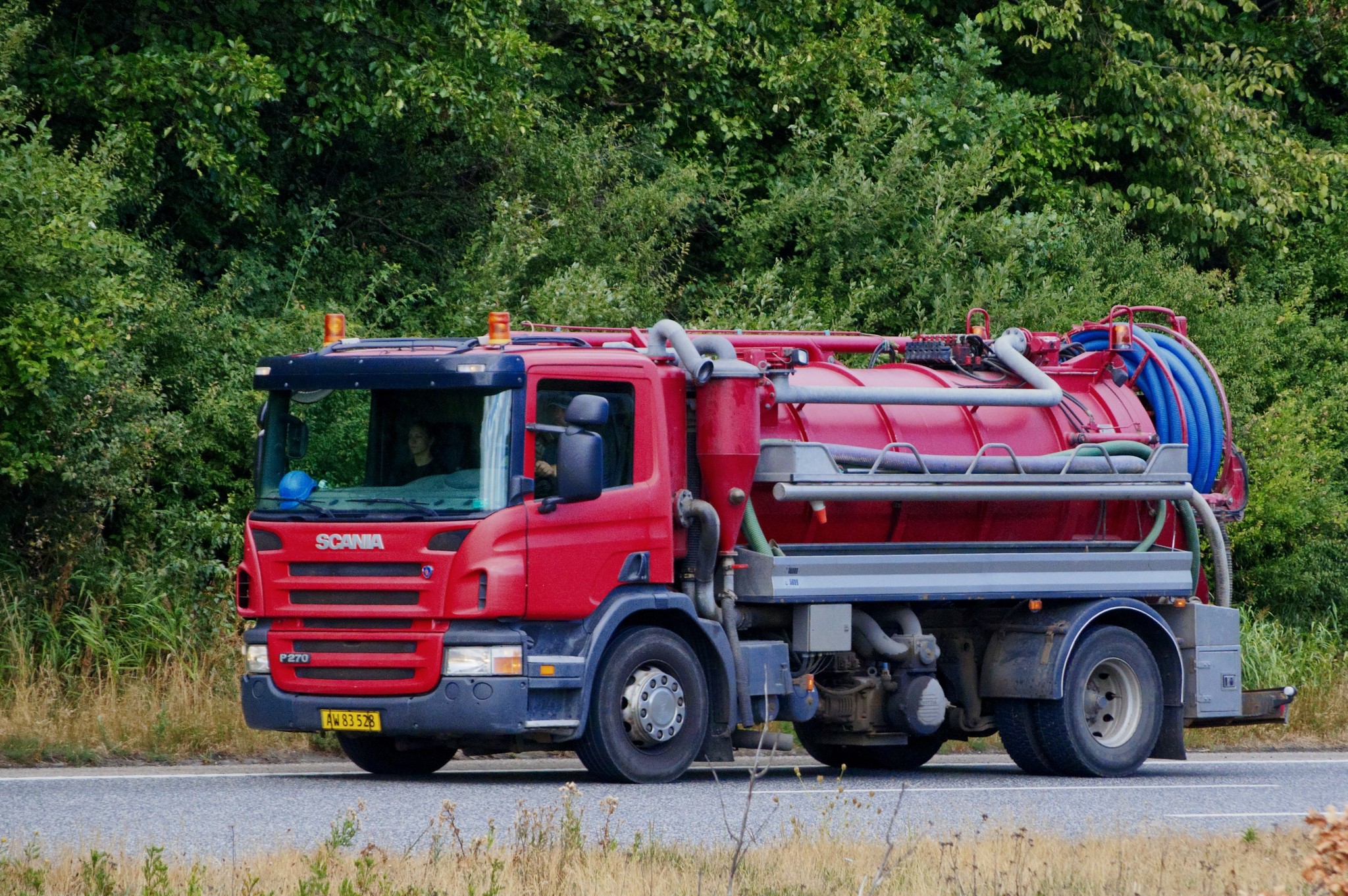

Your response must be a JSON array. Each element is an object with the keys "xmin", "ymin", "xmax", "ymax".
[
  {"xmin": 534, "ymin": 395, "xmax": 572, "ymax": 499},
  {"xmin": 387, "ymin": 422, "xmax": 448, "ymax": 486}
]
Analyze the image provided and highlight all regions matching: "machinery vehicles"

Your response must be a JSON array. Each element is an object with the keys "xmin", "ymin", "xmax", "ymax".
[{"xmin": 228, "ymin": 307, "xmax": 1294, "ymax": 784}]
